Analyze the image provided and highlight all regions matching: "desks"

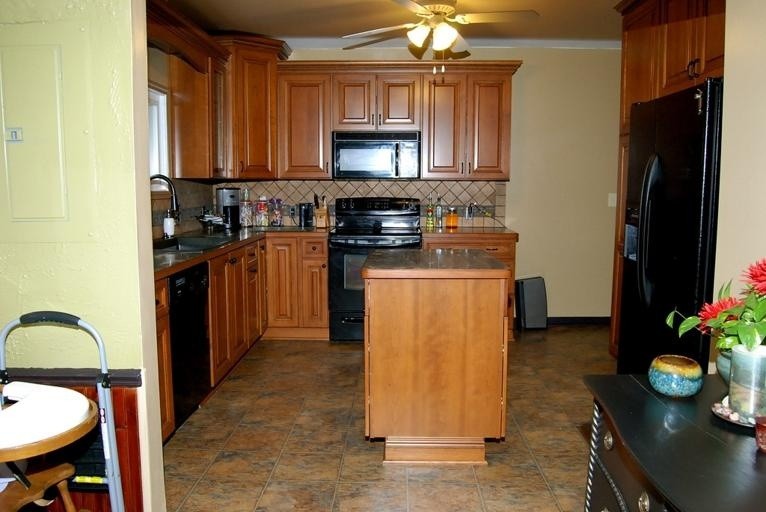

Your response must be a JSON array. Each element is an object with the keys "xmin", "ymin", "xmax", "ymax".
[{"xmin": 1, "ymin": 380, "xmax": 102, "ymax": 512}]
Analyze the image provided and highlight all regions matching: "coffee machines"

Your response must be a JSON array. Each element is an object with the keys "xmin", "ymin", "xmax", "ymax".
[{"xmin": 215, "ymin": 186, "xmax": 242, "ymax": 232}]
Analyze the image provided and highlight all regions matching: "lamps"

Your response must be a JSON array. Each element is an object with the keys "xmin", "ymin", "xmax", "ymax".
[{"xmin": 404, "ymin": 15, "xmax": 458, "ymax": 76}]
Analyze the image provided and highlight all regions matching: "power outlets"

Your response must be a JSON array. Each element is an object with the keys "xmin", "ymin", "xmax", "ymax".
[
  {"xmin": 289, "ymin": 205, "xmax": 296, "ymax": 219},
  {"xmin": 465, "ymin": 206, "xmax": 474, "ymax": 220}
]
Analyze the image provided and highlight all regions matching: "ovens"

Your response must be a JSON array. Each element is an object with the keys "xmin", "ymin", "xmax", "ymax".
[{"xmin": 327, "ymin": 245, "xmax": 376, "ymax": 344}]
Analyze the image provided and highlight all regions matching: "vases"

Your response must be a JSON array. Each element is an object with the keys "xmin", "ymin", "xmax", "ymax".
[{"xmin": 716, "ymin": 344, "xmax": 734, "ymax": 384}]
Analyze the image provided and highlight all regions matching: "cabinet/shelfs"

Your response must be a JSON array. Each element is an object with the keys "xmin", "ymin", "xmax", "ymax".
[
  {"xmin": 362, "ymin": 246, "xmax": 509, "ymax": 468},
  {"xmin": 246, "ymin": 238, "xmax": 265, "ymax": 350},
  {"xmin": 608, "ymin": 133, "xmax": 634, "ymax": 358},
  {"xmin": 231, "ymin": 40, "xmax": 280, "ymax": 180},
  {"xmin": 660, "ymin": 0, "xmax": 726, "ymax": 92},
  {"xmin": 208, "ymin": 247, "xmax": 248, "ymax": 389},
  {"xmin": 621, "ymin": 1, "xmax": 666, "ymax": 136},
  {"xmin": 422, "ymin": 67, "xmax": 512, "ymax": 180},
  {"xmin": 426, "ymin": 231, "xmax": 514, "ymax": 342},
  {"xmin": 265, "ymin": 231, "xmax": 327, "ymax": 329},
  {"xmin": 173, "ymin": 49, "xmax": 229, "ymax": 179},
  {"xmin": 581, "ymin": 365, "xmax": 766, "ymax": 512},
  {"xmin": 330, "ymin": 69, "xmax": 419, "ymax": 131},
  {"xmin": 153, "ymin": 277, "xmax": 176, "ymax": 445},
  {"xmin": 278, "ymin": 65, "xmax": 333, "ymax": 179}
]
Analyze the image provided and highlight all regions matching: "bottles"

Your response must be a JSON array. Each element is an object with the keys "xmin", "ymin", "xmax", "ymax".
[
  {"xmin": 464, "ymin": 203, "xmax": 475, "ymax": 221},
  {"xmin": 446, "ymin": 204, "xmax": 458, "ymax": 229},
  {"xmin": 237, "ymin": 189, "xmax": 284, "ymax": 228},
  {"xmin": 426, "ymin": 226, "xmax": 458, "ymax": 234},
  {"xmin": 426, "ymin": 196, "xmax": 433, "ymax": 228},
  {"xmin": 435, "ymin": 193, "xmax": 443, "ymax": 227}
]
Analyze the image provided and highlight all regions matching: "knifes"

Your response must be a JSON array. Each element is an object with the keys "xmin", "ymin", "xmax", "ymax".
[{"xmin": 311, "ymin": 192, "xmax": 328, "ymax": 208}]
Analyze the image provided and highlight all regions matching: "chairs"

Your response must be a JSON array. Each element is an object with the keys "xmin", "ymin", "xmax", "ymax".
[{"xmin": 0, "ymin": 309, "xmax": 127, "ymax": 512}]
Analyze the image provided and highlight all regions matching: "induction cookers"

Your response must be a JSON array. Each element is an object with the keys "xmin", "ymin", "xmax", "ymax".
[{"xmin": 327, "ymin": 226, "xmax": 422, "ymax": 249}]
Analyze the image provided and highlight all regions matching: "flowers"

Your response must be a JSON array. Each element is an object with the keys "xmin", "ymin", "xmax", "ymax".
[{"xmin": 667, "ymin": 256, "xmax": 765, "ymax": 359}]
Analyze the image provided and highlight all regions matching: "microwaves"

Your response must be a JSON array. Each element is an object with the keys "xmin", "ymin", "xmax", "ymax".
[{"xmin": 330, "ymin": 129, "xmax": 421, "ymax": 183}]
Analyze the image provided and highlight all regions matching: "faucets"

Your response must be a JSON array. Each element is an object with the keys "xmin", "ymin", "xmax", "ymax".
[{"xmin": 150, "ymin": 174, "xmax": 179, "ymax": 211}]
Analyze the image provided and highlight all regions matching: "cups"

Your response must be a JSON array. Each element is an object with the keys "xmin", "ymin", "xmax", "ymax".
[{"xmin": 729, "ymin": 345, "xmax": 766, "ymax": 419}]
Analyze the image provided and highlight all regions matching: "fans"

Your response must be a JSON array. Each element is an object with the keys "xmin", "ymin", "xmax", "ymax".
[{"xmin": 339, "ymin": 1, "xmax": 539, "ymax": 58}]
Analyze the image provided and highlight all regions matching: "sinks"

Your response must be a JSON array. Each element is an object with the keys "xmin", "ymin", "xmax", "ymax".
[{"xmin": 153, "ymin": 236, "xmax": 231, "ymax": 251}]
